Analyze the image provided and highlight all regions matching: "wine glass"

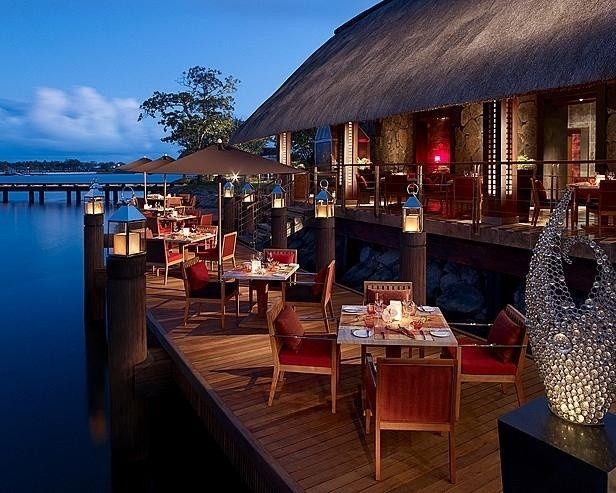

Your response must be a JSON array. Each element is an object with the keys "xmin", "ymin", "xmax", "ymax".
[{"xmin": 172, "ymin": 221, "xmax": 197, "ymax": 233}]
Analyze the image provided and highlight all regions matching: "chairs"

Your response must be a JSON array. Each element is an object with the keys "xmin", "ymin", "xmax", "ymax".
[
  {"xmin": 438, "ymin": 304, "xmax": 531, "ymax": 408},
  {"xmin": 265, "ymin": 297, "xmax": 341, "ymax": 414},
  {"xmin": 132, "ymin": 191, "xmax": 212, "ymax": 233},
  {"xmin": 353, "ymin": 162, "xmax": 616, "ymax": 236},
  {"xmin": 363, "ymin": 354, "xmax": 458, "ymax": 486},
  {"xmin": 180, "ymin": 248, "xmax": 336, "ymax": 333},
  {"xmin": 361, "ymin": 281, "xmax": 414, "ymax": 369},
  {"xmin": 141, "ymin": 224, "xmax": 237, "ymax": 285}
]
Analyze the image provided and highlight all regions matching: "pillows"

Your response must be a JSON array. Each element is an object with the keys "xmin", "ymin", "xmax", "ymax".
[
  {"xmin": 273, "ymin": 303, "xmax": 305, "ymax": 353},
  {"xmin": 487, "ymin": 308, "xmax": 523, "ymax": 364},
  {"xmin": 365, "ymin": 289, "xmax": 412, "ymax": 306}
]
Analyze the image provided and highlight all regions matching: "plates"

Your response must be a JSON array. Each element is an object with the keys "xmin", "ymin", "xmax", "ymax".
[
  {"xmin": 243, "ymin": 261, "xmax": 290, "ymax": 277},
  {"xmin": 344, "ymin": 304, "xmax": 451, "ymax": 339}
]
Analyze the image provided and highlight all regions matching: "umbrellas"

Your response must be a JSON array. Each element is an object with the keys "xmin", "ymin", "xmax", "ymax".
[
  {"xmin": 116, "ymin": 156, "xmax": 152, "ymax": 204},
  {"xmin": 147, "ymin": 139, "xmax": 308, "ymax": 282},
  {"xmin": 125, "ymin": 154, "xmax": 176, "ymax": 216}
]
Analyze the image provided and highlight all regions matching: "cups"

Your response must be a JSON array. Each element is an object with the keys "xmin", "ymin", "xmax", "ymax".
[
  {"xmin": 605, "ymin": 168, "xmax": 615, "ymax": 180},
  {"xmin": 250, "ymin": 250, "xmax": 274, "ymax": 263},
  {"xmin": 594, "ymin": 171, "xmax": 599, "ymax": 178}
]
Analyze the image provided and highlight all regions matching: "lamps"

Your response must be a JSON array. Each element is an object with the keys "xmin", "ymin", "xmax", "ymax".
[
  {"xmin": 106, "ymin": 199, "xmax": 146, "ymax": 258},
  {"xmin": 313, "ymin": 178, "xmax": 338, "ymax": 217},
  {"xmin": 82, "ymin": 174, "xmax": 104, "ymax": 215},
  {"xmin": 272, "ymin": 174, "xmax": 289, "ymax": 208},
  {"xmin": 240, "ymin": 179, "xmax": 257, "ymax": 202},
  {"xmin": 223, "ymin": 176, "xmax": 235, "ymax": 199}
]
